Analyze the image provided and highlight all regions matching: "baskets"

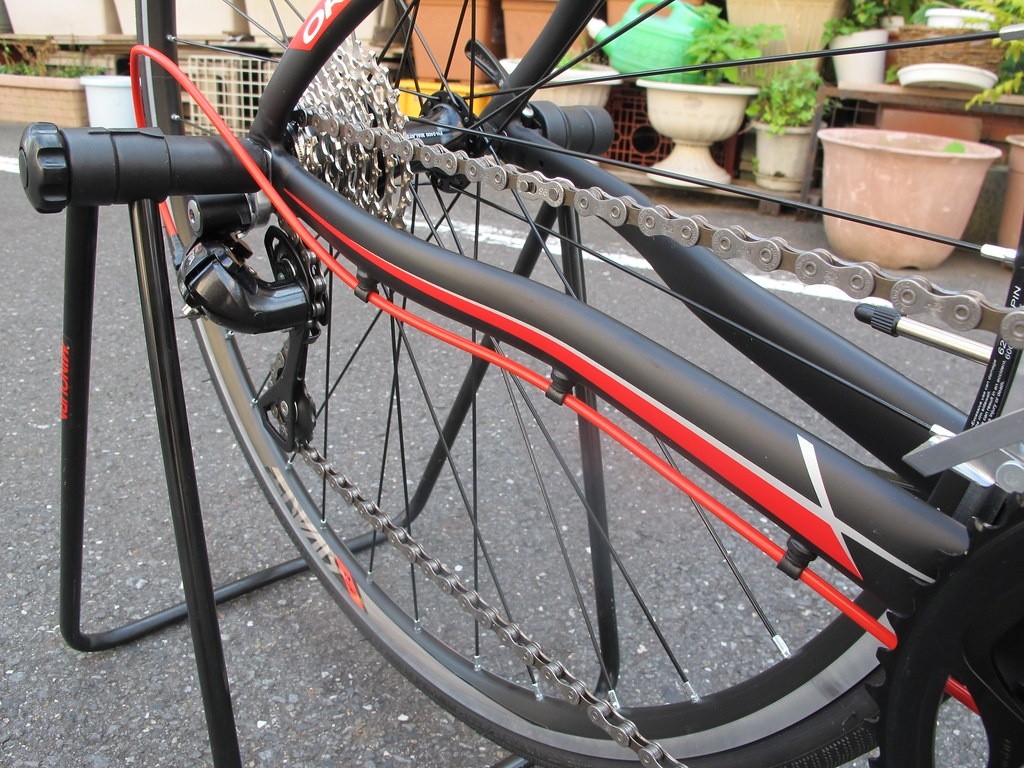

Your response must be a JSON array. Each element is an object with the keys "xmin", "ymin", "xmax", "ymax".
[{"xmin": 898, "ymin": 24, "xmax": 1012, "ymax": 79}]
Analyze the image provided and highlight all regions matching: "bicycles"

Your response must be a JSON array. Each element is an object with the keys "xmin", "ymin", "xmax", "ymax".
[{"xmin": 18, "ymin": 1, "xmax": 1022, "ymax": 768}]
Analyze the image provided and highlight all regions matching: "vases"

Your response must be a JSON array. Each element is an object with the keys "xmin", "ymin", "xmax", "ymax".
[
  {"xmin": 888, "ymin": 24, "xmax": 1009, "ymax": 93},
  {"xmin": 922, "ymin": 6, "xmax": 998, "ymax": 44},
  {"xmin": 812, "ymin": 121, "xmax": 1003, "ymax": 272},
  {"xmin": 998, "ymin": 130, "xmax": 1024, "ymax": 261}
]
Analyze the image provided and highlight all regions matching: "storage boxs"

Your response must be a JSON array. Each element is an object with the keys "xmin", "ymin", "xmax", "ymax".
[{"xmin": 75, "ymin": 43, "xmax": 502, "ymax": 157}]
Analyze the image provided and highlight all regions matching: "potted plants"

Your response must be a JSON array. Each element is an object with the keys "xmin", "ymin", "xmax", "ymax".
[
  {"xmin": 490, "ymin": 26, "xmax": 626, "ymax": 120},
  {"xmin": 2, "ymin": 29, "xmax": 114, "ymax": 126},
  {"xmin": 745, "ymin": 57, "xmax": 843, "ymax": 192},
  {"xmin": 635, "ymin": 1, "xmax": 787, "ymax": 190},
  {"xmin": 820, "ymin": 0, "xmax": 890, "ymax": 90}
]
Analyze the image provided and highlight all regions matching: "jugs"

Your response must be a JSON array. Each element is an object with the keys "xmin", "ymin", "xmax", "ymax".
[{"xmin": 584, "ymin": 0, "xmax": 717, "ymax": 90}]
